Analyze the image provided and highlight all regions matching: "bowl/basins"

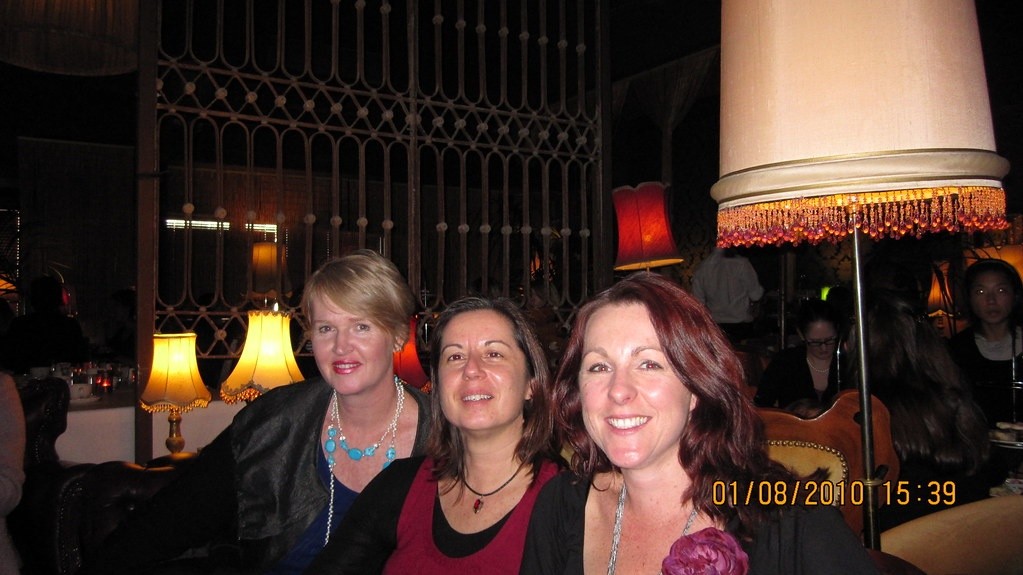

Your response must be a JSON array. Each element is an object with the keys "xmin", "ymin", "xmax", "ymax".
[{"xmin": 69, "ymin": 384, "xmax": 92, "ymax": 397}]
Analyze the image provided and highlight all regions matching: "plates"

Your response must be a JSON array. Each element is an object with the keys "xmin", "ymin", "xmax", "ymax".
[{"xmin": 68, "ymin": 397, "xmax": 100, "ymax": 407}]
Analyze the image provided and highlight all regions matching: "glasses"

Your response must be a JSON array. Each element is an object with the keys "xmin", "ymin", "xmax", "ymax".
[{"xmin": 801, "ymin": 330, "xmax": 838, "ymax": 346}]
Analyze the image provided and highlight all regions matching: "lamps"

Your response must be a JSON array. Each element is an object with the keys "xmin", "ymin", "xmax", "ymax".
[
  {"xmin": 220, "ymin": 309, "xmax": 305, "ymax": 406},
  {"xmin": 393, "ymin": 317, "xmax": 431, "ymax": 393},
  {"xmin": 709, "ymin": 1, "xmax": 1008, "ymax": 553},
  {"xmin": 610, "ymin": 183, "xmax": 684, "ymax": 273},
  {"xmin": 139, "ymin": 333, "xmax": 211, "ymax": 464}
]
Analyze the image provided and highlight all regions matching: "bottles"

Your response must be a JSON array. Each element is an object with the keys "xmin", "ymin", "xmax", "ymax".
[{"xmin": 48, "ymin": 361, "xmax": 136, "ymax": 392}]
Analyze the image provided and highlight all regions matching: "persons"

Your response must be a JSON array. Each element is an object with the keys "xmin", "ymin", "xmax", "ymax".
[
  {"xmin": 692, "ymin": 245, "xmax": 764, "ymax": 341},
  {"xmin": 753, "ymin": 298, "xmax": 856, "ymax": 410},
  {"xmin": 5, "ymin": 263, "xmax": 89, "ymax": 374},
  {"xmin": 519, "ymin": 271, "xmax": 883, "ymax": 575},
  {"xmin": 301, "ymin": 294, "xmax": 569, "ymax": 575},
  {"xmin": 945, "ymin": 258, "xmax": 1023, "ymax": 423},
  {"xmin": 0, "ymin": 372, "xmax": 26, "ymax": 575},
  {"xmin": 846, "ymin": 288, "xmax": 991, "ymax": 532},
  {"xmin": 229, "ymin": 248, "xmax": 431, "ymax": 575}
]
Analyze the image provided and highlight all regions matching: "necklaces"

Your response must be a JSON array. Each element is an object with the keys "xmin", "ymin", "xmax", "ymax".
[
  {"xmin": 607, "ymin": 483, "xmax": 697, "ymax": 575},
  {"xmin": 465, "ymin": 463, "xmax": 523, "ymax": 514},
  {"xmin": 324, "ymin": 374, "xmax": 405, "ymax": 546},
  {"xmin": 806, "ymin": 358, "xmax": 829, "ymax": 372}
]
